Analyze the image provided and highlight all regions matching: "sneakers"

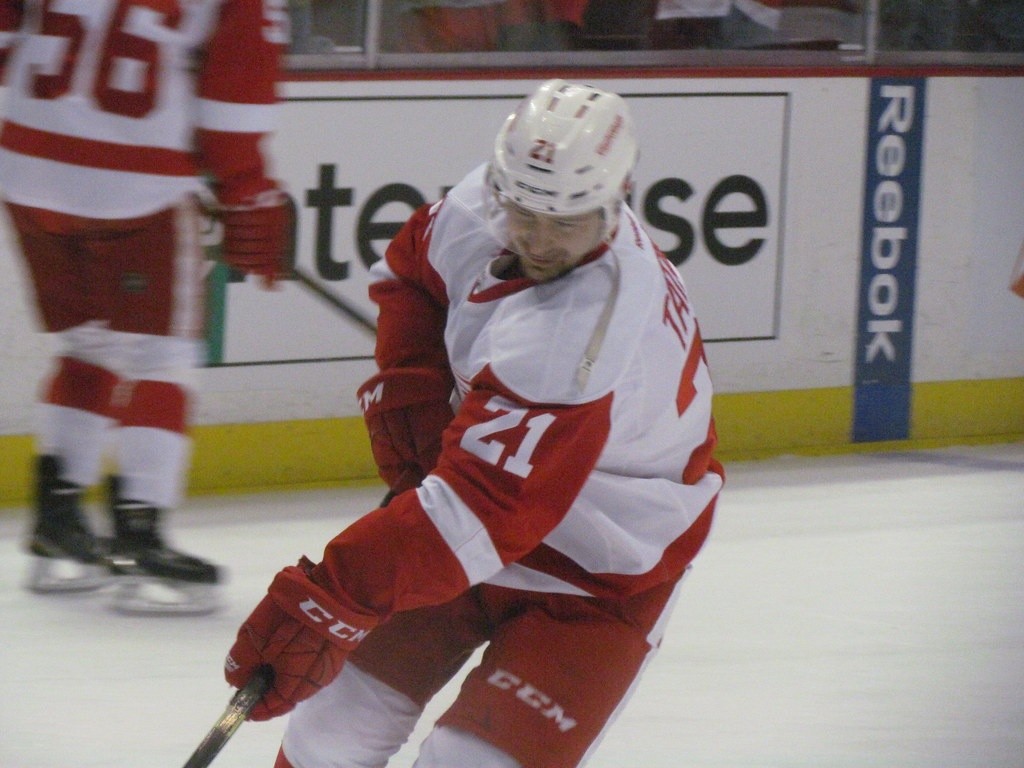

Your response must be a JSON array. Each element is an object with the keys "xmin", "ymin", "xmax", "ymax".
[
  {"xmin": 105, "ymin": 505, "xmax": 218, "ymax": 613},
  {"xmin": 19, "ymin": 455, "xmax": 120, "ymax": 589}
]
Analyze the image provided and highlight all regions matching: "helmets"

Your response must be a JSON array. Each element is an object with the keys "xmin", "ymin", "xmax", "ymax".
[{"xmin": 492, "ymin": 77, "xmax": 638, "ymax": 216}]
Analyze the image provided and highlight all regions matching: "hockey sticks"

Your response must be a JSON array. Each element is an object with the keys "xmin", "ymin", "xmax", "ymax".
[
  {"xmin": 182, "ymin": 667, "xmax": 271, "ymax": 768},
  {"xmin": 196, "ymin": 195, "xmax": 379, "ymax": 338}
]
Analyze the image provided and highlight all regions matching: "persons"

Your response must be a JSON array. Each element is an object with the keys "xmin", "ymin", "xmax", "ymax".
[
  {"xmin": 0, "ymin": 0, "xmax": 298, "ymax": 616},
  {"xmin": 378, "ymin": 0, "xmax": 782, "ymax": 53},
  {"xmin": 223, "ymin": 79, "xmax": 727, "ymax": 767}
]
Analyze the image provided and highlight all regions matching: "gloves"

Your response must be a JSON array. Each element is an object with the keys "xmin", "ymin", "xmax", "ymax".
[
  {"xmin": 225, "ymin": 558, "xmax": 380, "ymax": 720},
  {"xmin": 221, "ymin": 186, "xmax": 293, "ymax": 275},
  {"xmin": 356, "ymin": 368, "xmax": 456, "ymax": 492}
]
